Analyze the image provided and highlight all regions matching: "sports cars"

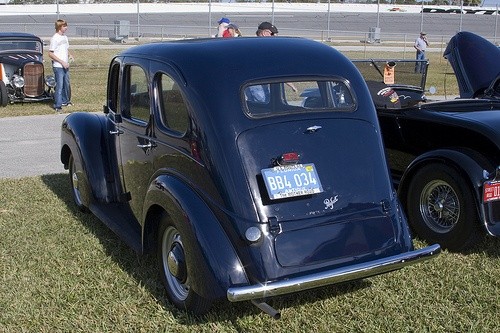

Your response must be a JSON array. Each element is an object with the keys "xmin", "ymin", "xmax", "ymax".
[{"xmin": 300, "ymin": 55, "xmax": 500, "ymax": 251}]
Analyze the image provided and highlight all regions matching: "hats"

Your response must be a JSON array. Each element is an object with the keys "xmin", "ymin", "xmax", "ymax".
[
  {"xmin": 420, "ymin": 32, "xmax": 427, "ymax": 35},
  {"xmin": 223, "ymin": 24, "xmax": 239, "ymax": 29},
  {"xmin": 217, "ymin": 17, "xmax": 230, "ymax": 24},
  {"xmin": 258, "ymin": 22, "xmax": 278, "ymax": 33}
]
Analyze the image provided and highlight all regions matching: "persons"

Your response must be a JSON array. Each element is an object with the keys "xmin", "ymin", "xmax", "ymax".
[
  {"xmin": 414, "ymin": 32, "xmax": 429, "ymax": 73},
  {"xmin": 245, "ymin": 22, "xmax": 296, "ymax": 102},
  {"xmin": 49, "ymin": 19, "xmax": 74, "ymax": 111},
  {"xmin": 215, "ymin": 18, "xmax": 241, "ymax": 38}
]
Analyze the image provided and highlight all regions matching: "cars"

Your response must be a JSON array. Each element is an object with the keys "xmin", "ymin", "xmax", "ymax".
[
  {"xmin": 0, "ymin": 31, "xmax": 57, "ymax": 106},
  {"xmin": 71, "ymin": 35, "xmax": 444, "ymax": 321}
]
